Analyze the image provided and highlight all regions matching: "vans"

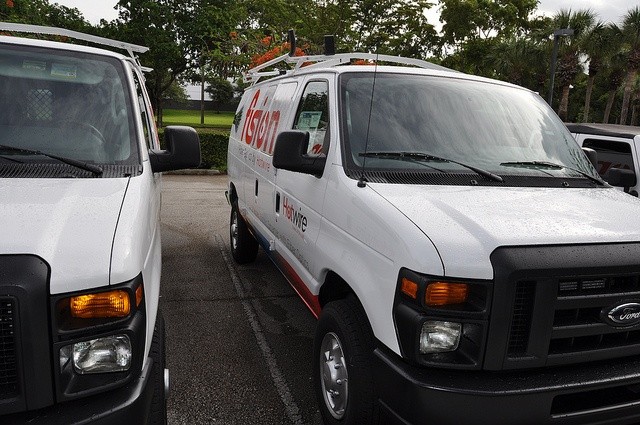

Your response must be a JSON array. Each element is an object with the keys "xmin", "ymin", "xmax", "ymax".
[
  {"xmin": 563, "ymin": 120, "xmax": 640, "ymax": 198},
  {"xmin": 225, "ymin": 47, "xmax": 640, "ymax": 424},
  {"xmin": 0, "ymin": 15, "xmax": 176, "ymax": 424}
]
